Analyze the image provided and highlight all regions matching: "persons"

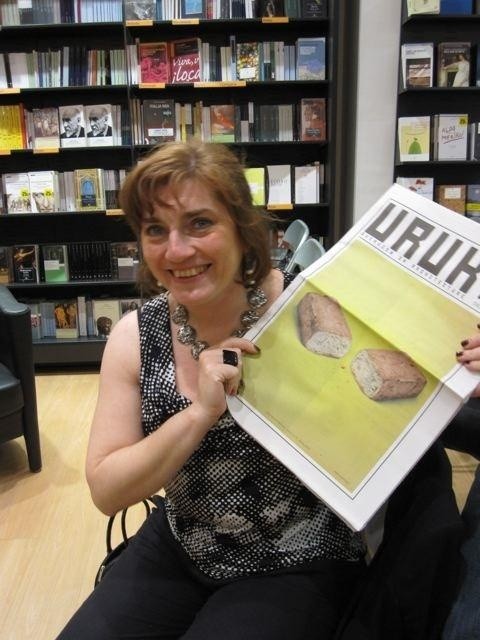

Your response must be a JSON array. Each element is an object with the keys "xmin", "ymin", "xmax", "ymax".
[{"xmin": 56, "ymin": 142, "xmax": 480, "ymax": 640}]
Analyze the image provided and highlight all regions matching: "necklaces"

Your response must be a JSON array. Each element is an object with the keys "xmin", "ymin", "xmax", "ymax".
[{"xmin": 171, "ymin": 287, "xmax": 268, "ymax": 361}]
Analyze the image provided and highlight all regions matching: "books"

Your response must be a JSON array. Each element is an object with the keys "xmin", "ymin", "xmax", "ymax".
[
  {"xmin": 1, "ymin": 1, "xmax": 330, "ymax": 338},
  {"xmin": 395, "ymin": 0, "xmax": 480, "ymax": 217}
]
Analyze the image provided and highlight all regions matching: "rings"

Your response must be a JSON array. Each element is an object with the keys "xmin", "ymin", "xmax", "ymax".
[{"xmin": 222, "ymin": 350, "xmax": 238, "ymax": 366}]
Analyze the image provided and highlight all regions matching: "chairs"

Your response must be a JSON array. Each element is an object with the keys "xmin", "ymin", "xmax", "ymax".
[{"xmin": 0, "ymin": 279, "xmax": 44, "ymax": 473}]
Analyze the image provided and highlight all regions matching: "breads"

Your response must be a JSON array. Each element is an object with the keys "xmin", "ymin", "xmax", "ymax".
[
  {"xmin": 351, "ymin": 349, "xmax": 428, "ymax": 401},
  {"xmin": 296, "ymin": 292, "xmax": 352, "ymax": 358}
]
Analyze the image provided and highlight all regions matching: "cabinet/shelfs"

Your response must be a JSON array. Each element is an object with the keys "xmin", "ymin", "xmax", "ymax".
[
  {"xmin": 0, "ymin": 1, "xmax": 349, "ymax": 369},
  {"xmin": 395, "ymin": 10, "xmax": 480, "ymax": 180}
]
